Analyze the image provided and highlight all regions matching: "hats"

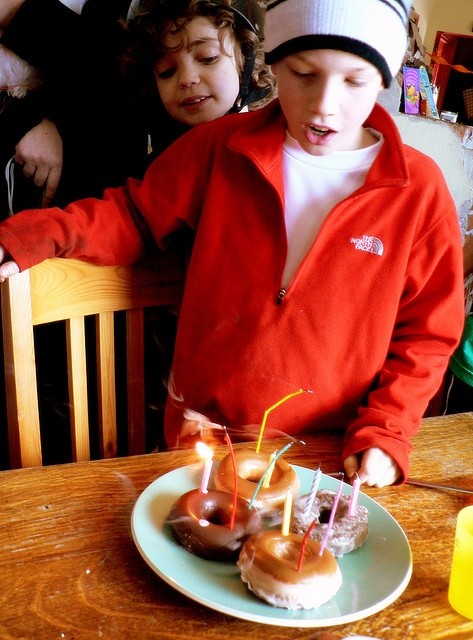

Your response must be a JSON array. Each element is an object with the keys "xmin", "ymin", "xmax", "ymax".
[{"xmin": 264, "ymin": 0, "xmax": 414, "ymax": 88}]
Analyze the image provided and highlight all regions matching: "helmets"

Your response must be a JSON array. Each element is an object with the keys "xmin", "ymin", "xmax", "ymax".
[{"xmin": 214, "ymin": 1, "xmax": 262, "ymax": 38}]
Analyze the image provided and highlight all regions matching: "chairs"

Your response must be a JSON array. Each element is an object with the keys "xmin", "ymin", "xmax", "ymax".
[{"xmin": 0, "ymin": 236, "xmax": 195, "ymax": 470}]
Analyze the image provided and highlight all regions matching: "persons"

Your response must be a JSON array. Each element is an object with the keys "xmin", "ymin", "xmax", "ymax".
[
  {"xmin": 0, "ymin": 1, "xmax": 466, "ymax": 489},
  {"xmin": 0, "ymin": 0, "xmax": 121, "ymax": 214},
  {"xmin": 117, "ymin": 1, "xmax": 279, "ymax": 183}
]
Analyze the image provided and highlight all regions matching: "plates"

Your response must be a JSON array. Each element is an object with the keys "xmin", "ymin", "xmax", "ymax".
[{"xmin": 128, "ymin": 463, "xmax": 413, "ymax": 628}]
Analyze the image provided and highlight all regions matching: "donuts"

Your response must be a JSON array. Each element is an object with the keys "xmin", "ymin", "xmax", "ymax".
[
  {"xmin": 171, "ymin": 484, "xmax": 262, "ymax": 561},
  {"xmin": 213, "ymin": 446, "xmax": 299, "ymax": 514},
  {"xmin": 286, "ymin": 487, "xmax": 369, "ymax": 557},
  {"xmin": 237, "ymin": 529, "xmax": 344, "ymax": 610}
]
